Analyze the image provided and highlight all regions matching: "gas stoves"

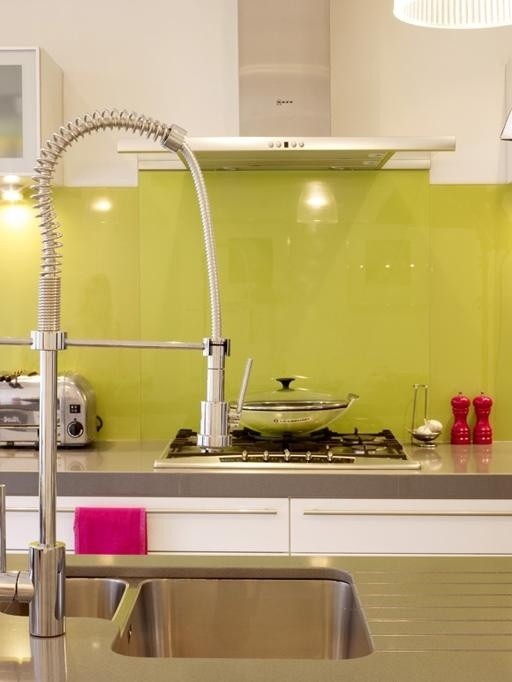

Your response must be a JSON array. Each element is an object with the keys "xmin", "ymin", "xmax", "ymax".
[{"xmin": 153, "ymin": 424, "xmax": 419, "ymax": 469}]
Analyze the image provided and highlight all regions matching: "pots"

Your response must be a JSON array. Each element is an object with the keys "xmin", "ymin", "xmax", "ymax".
[{"xmin": 226, "ymin": 377, "xmax": 359, "ymax": 439}]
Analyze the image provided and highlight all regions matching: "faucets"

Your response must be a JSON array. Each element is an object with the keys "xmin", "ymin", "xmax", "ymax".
[{"xmin": 0, "ymin": 108, "xmax": 233, "ymax": 636}]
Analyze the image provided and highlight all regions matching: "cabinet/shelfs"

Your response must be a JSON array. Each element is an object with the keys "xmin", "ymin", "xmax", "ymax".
[
  {"xmin": 0, "ymin": 43, "xmax": 67, "ymax": 189},
  {"xmin": 0, "ymin": 476, "xmax": 512, "ymax": 557}
]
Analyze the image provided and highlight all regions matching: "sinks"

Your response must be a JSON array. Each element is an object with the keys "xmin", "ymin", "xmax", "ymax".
[
  {"xmin": 111, "ymin": 565, "xmax": 374, "ymax": 659},
  {"xmin": 0, "ymin": 566, "xmax": 130, "ymax": 622}
]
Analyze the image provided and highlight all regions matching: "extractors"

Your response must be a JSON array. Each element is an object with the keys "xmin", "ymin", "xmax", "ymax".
[{"xmin": 116, "ymin": 0, "xmax": 456, "ymax": 172}]
